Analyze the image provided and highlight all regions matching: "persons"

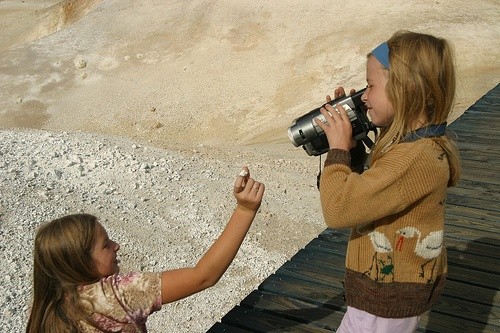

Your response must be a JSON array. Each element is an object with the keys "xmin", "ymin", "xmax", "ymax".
[
  {"xmin": 319, "ymin": 29, "xmax": 463, "ymax": 333},
  {"xmin": 26, "ymin": 167, "xmax": 265, "ymax": 333}
]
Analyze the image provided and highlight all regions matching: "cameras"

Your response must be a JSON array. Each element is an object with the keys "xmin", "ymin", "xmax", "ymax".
[{"xmin": 287, "ymin": 88, "xmax": 376, "ymax": 157}]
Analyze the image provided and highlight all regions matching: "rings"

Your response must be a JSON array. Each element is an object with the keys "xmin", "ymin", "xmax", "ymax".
[{"xmin": 252, "ymin": 187, "xmax": 258, "ymax": 190}]
